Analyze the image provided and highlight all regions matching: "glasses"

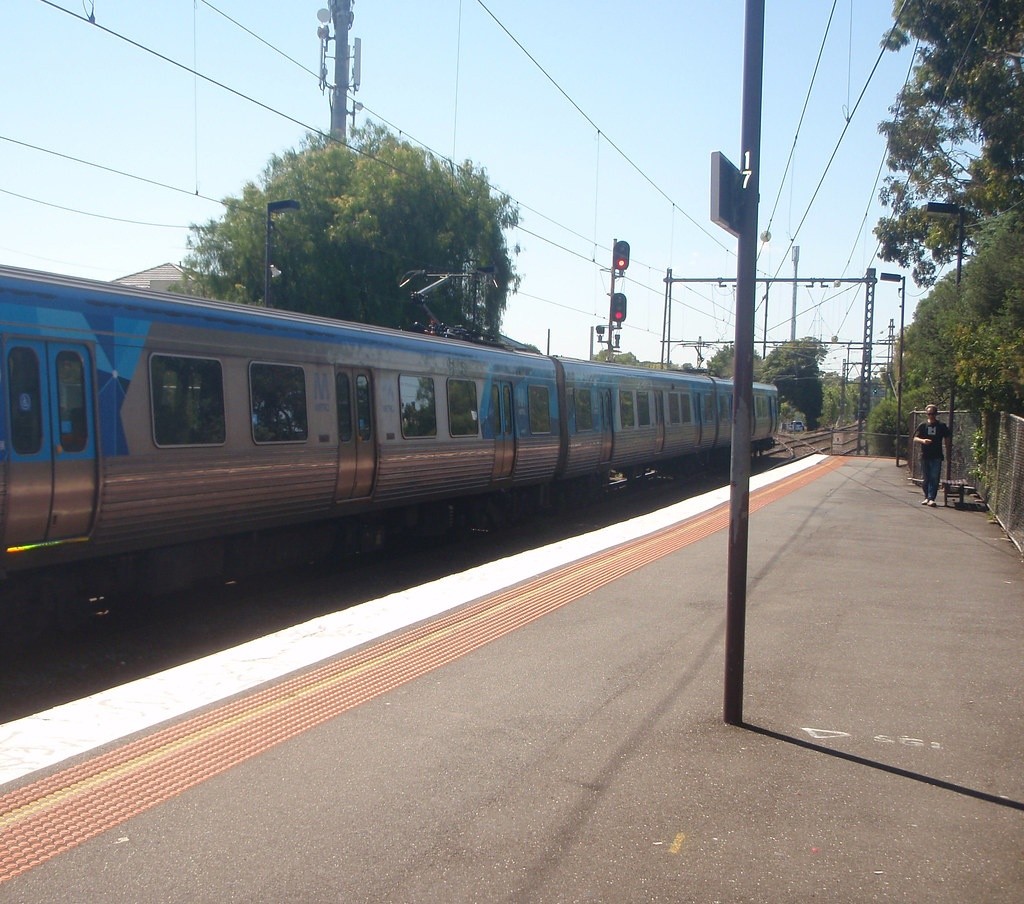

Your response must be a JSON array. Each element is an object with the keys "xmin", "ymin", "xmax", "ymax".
[{"xmin": 926, "ymin": 412, "xmax": 937, "ymax": 415}]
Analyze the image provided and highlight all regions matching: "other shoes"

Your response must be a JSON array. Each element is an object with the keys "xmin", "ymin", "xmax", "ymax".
[
  {"xmin": 927, "ymin": 500, "xmax": 936, "ymax": 506},
  {"xmin": 922, "ymin": 499, "xmax": 930, "ymax": 506}
]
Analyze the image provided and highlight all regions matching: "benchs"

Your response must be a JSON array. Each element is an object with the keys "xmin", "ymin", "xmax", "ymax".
[{"xmin": 941, "ymin": 478, "xmax": 968, "ymax": 507}]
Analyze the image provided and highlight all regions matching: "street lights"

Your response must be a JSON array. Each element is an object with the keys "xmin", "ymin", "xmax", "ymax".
[
  {"xmin": 926, "ymin": 200, "xmax": 965, "ymax": 481},
  {"xmin": 791, "ymin": 245, "xmax": 800, "ymax": 343},
  {"xmin": 880, "ymin": 271, "xmax": 905, "ymax": 469}
]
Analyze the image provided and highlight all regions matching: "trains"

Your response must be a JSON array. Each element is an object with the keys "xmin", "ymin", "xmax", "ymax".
[{"xmin": 2, "ymin": 263, "xmax": 784, "ymax": 565}]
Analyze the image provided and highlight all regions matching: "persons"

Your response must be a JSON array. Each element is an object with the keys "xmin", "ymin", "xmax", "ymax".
[{"xmin": 911, "ymin": 405, "xmax": 952, "ymax": 507}]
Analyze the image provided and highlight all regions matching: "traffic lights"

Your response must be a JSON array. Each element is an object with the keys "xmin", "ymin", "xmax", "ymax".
[
  {"xmin": 613, "ymin": 240, "xmax": 630, "ymax": 270},
  {"xmin": 610, "ymin": 293, "xmax": 628, "ymax": 323}
]
